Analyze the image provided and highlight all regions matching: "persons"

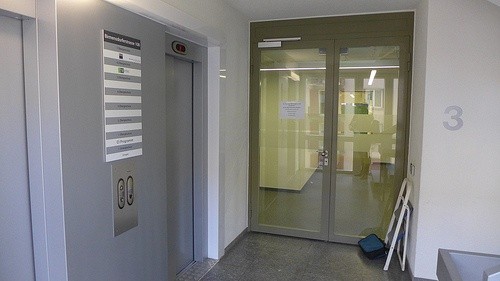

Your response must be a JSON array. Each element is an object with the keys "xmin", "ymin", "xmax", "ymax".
[{"xmin": 350, "ymin": 105, "xmax": 374, "ymax": 179}]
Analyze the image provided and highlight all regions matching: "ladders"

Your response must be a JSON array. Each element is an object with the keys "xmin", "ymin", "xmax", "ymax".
[{"xmin": 382, "ymin": 177, "xmax": 414, "ymax": 272}]
